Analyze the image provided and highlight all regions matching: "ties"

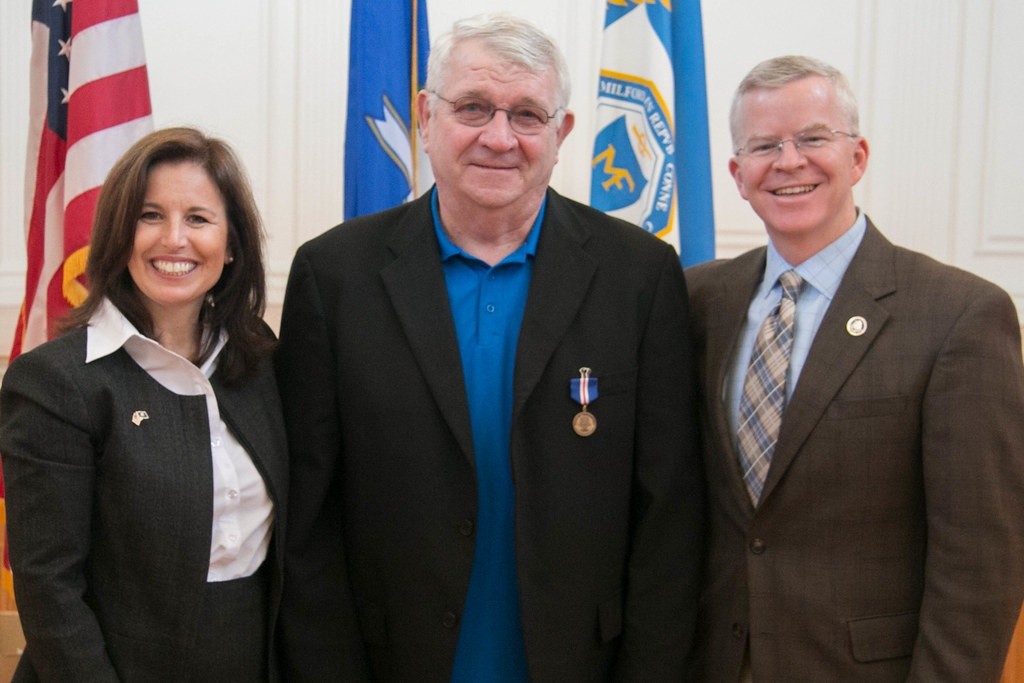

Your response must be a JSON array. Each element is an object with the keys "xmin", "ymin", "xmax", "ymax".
[{"xmin": 734, "ymin": 273, "xmax": 805, "ymax": 506}]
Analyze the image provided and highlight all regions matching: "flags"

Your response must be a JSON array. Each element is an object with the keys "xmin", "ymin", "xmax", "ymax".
[
  {"xmin": 589, "ymin": 0, "xmax": 716, "ymax": 268},
  {"xmin": 0, "ymin": 0, "xmax": 156, "ymax": 611},
  {"xmin": 345, "ymin": 0, "xmax": 436, "ymax": 224}
]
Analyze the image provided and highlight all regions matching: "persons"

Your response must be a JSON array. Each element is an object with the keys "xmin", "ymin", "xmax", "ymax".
[
  {"xmin": 279, "ymin": 13, "xmax": 708, "ymax": 683},
  {"xmin": 0, "ymin": 130, "xmax": 290, "ymax": 683},
  {"xmin": 683, "ymin": 54, "xmax": 1024, "ymax": 683}
]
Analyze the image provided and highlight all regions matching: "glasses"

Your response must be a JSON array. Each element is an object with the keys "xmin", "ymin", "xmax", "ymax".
[
  {"xmin": 733, "ymin": 120, "xmax": 855, "ymax": 161},
  {"xmin": 426, "ymin": 89, "xmax": 563, "ymax": 137}
]
